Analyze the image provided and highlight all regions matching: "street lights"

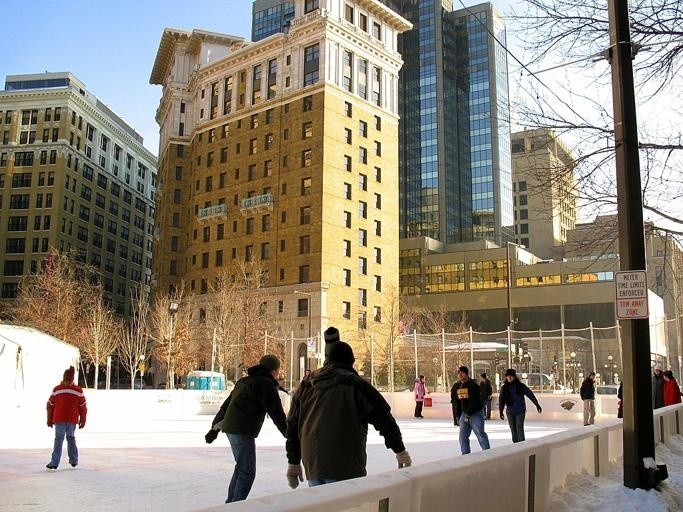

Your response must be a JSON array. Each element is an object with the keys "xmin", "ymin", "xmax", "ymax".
[
  {"xmin": 164, "ymin": 300, "xmax": 179, "ymax": 387},
  {"xmin": 292, "ymin": 287, "xmax": 312, "ymax": 375},
  {"xmin": 506, "ymin": 240, "xmax": 527, "ymax": 369},
  {"xmin": 566, "ymin": 352, "xmax": 581, "ymax": 393},
  {"xmin": 603, "ymin": 354, "xmax": 618, "ymax": 385}
]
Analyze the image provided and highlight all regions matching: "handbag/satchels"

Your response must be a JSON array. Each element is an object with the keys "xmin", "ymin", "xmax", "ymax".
[{"xmin": 423, "ymin": 397, "xmax": 433, "ymax": 408}]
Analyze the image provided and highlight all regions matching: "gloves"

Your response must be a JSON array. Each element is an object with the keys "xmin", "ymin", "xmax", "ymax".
[
  {"xmin": 286, "ymin": 464, "xmax": 304, "ymax": 490},
  {"xmin": 395, "ymin": 450, "xmax": 413, "ymax": 470},
  {"xmin": 205, "ymin": 428, "xmax": 219, "ymax": 445},
  {"xmin": 79, "ymin": 422, "xmax": 84, "ymax": 429},
  {"xmin": 47, "ymin": 421, "xmax": 54, "ymax": 428}
]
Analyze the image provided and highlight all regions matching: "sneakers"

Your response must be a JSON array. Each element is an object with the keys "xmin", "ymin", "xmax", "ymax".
[
  {"xmin": 71, "ymin": 464, "xmax": 78, "ymax": 467},
  {"xmin": 484, "ymin": 415, "xmax": 490, "ymax": 419},
  {"xmin": 46, "ymin": 464, "xmax": 57, "ymax": 470}
]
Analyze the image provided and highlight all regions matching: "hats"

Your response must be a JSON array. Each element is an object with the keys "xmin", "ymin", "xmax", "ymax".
[
  {"xmin": 260, "ymin": 355, "xmax": 280, "ymax": 370},
  {"xmin": 654, "ymin": 364, "xmax": 662, "ymax": 370},
  {"xmin": 480, "ymin": 373, "xmax": 486, "ymax": 378},
  {"xmin": 326, "ymin": 340, "xmax": 356, "ymax": 366},
  {"xmin": 505, "ymin": 369, "xmax": 517, "ymax": 377},
  {"xmin": 457, "ymin": 366, "xmax": 468, "ymax": 375},
  {"xmin": 663, "ymin": 371, "xmax": 672, "ymax": 375},
  {"xmin": 63, "ymin": 366, "xmax": 74, "ymax": 382},
  {"xmin": 537, "ymin": 405, "xmax": 542, "ymax": 414},
  {"xmin": 500, "ymin": 412, "xmax": 505, "ymax": 420}
]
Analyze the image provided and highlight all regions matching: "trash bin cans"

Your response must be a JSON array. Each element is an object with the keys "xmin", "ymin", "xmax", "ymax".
[{"xmin": 492, "ymin": 396, "xmax": 500, "ymax": 411}]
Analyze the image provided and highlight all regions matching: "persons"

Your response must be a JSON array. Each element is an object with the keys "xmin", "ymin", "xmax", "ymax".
[
  {"xmin": 479, "ymin": 372, "xmax": 492, "ymax": 420},
  {"xmin": 616, "ymin": 380, "xmax": 623, "ymax": 418},
  {"xmin": 450, "ymin": 380, "xmax": 462, "ymax": 426},
  {"xmin": 579, "ymin": 371, "xmax": 596, "ymax": 426},
  {"xmin": 661, "ymin": 370, "xmax": 680, "ymax": 406},
  {"xmin": 285, "ymin": 341, "xmax": 413, "ymax": 489},
  {"xmin": 652, "ymin": 365, "xmax": 666, "ymax": 409},
  {"xmin": 45, "ymin": 365, "xmax": 87, "ymax": 469},
  {"xmin": 203, "ymin": 353, "xmax": 287, "ymax": 503},
  {"xmin": 498, "ymin": 369, "xmax": 542, "ymax": 443},
  {"xmin": 413, "ymin": 374, "xmax": 426, "ymax": 418},
  {"xmin": 452, "ymin": 365, "xmax": 489, "ymax": 455}
]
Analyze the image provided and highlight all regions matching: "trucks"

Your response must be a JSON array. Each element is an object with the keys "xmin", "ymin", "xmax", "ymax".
[{"xmin": 516, "ymin": 372, "xmax": 565, "ymax": 391}]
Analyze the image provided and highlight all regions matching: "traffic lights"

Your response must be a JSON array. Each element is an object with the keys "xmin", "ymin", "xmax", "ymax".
[
  {"xmin": 523, "ymin": 342, "xmax": 528, "ymax": 355},
  {"xmin": 515, "ymin": 357, "xmax": 520, "ymax": 364}
]
